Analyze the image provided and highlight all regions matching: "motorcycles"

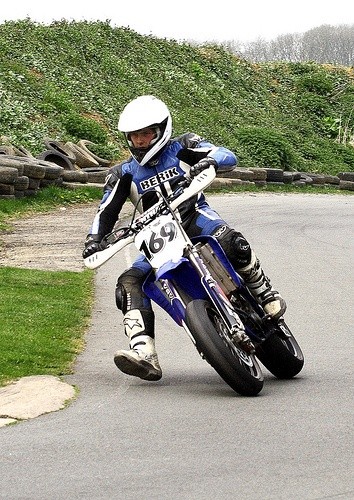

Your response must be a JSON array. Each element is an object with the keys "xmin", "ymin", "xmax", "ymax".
[{"xmin": 82, "ymin": 165, "xmax": 305, "ymax": 397}]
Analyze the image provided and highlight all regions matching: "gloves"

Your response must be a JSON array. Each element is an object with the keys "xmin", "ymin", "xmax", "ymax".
[
  {"xmin": 82, "ymin": 234, "xmax": 107, "ymax": 259},
  {"xmin": 189, "ymin": 156, "xmax": 218, "ymax": 179}
]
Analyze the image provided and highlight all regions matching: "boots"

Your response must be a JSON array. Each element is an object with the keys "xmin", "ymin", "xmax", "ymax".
[
  {"xmin": 235, "ymin": 248, "xmax": 286, "ymax": 319},
  {"xmin": 114, "ymin": 309, "xmax": 162, "ymax": 381}
]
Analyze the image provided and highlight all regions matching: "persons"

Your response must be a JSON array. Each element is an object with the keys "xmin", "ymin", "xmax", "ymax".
[{"xmin": 82, "ymin": 95, "xmax": 287, "ymax": 381}]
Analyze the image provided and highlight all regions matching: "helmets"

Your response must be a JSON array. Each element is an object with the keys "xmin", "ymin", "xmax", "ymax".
[{"xmin": 118, "ymin": 96, "xmax": 172, "ymax": 166}]
